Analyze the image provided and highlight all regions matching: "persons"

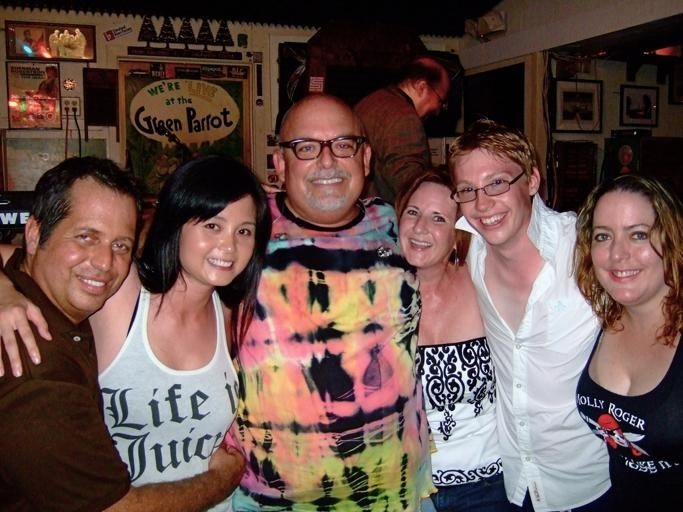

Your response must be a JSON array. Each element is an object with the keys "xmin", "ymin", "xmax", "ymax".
[
  {"xmin": 20, "ymin": 28, "xmax": 35, "ymax": 54},
  {"xmin": 216, "ymin": 89, "xmax": 441, "ymax": 512},
  {"xmin": 37, "ymin": 64, "xmax": 59, "ymax": 99},
  {"xmin": 571, "ymin": 170, "xmax": 682, "ymax": 512},
  {"xmin": 394, "ymin": 164, "xmax": 514, "ymax": 512},
  {"xmin": 49, "ymin": 28, "xmax": 87, "ymax": 59},
  {"xmin": 353, "ymin": 54, "xmax": 453, "ymax": 208},
  {"xmin": 447, "ymin": 115, "xmax": 614, "ymax": 511}
]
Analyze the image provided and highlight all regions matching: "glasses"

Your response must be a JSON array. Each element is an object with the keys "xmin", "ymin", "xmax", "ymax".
[
  {"xmin": 279, "ymin": 135, "xmax": 366, "ymax": 159},
  {"xmin": 451, "ymin": 170, "xmax": 525, "ymax": 203}
]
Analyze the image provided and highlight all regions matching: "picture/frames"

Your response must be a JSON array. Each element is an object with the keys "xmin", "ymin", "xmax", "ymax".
[
  {"xmin": 61, "ymin": 98, "xmax": 81, "ymax": 116},
  {"xmin": 549, "ymin": 79, "xmax": 603, "ymax": 134},
  {"xmin": 620, "ymin": 85, "xmax": 659, "ymax": 128}
]
